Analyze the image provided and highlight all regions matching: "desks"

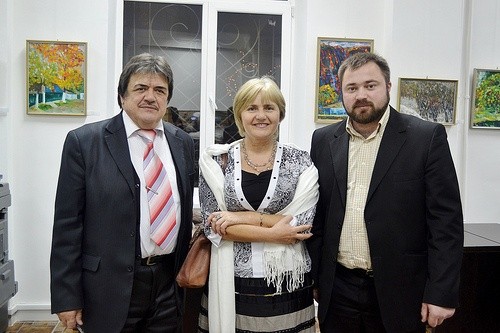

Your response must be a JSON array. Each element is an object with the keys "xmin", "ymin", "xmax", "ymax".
[{"xmin": 464, "ymin": 223, "xmax": 500, "ymax": 251}]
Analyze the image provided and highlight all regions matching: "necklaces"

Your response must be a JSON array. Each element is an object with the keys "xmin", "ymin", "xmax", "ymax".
[{"xmin": 241, "ymin": 139, "xmax": 276, "ymax": 173}]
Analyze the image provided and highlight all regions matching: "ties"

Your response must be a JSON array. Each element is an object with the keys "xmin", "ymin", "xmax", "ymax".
[{"xmin": 134, "ymin": 128, "xmax": 177, "ymax": 251}]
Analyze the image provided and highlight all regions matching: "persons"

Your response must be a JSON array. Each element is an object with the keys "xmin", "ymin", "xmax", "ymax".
[
  {"xmin": 49, "ymin": 53, "xmax": 195, "ymax": 333},
  {"xmin": 199, "ymin": 76, "xmax": 319, "ymax": 333},
  {"xmin": 310, "ymin": 52, "xmax": 464, "ymax": 333}
]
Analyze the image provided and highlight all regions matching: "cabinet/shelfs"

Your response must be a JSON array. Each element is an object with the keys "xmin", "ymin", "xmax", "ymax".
[{"xmin": 0, "ymin": 175, "xmax": 18, "ymax": 309}]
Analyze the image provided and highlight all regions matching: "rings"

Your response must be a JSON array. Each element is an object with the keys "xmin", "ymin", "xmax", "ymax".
[{"xmin": 215, "ymin": 214, "xmax": 221, "ymax": 220}]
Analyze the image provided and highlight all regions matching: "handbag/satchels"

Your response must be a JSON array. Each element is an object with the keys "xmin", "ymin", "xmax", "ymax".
[{"xmin": 175, "ymin": 221, "xmax": 212, "ymax": 289}]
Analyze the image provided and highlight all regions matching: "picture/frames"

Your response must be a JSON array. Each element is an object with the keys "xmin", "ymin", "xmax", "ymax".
[
  {"xmin": 397, "ymin": 78, "xmax": 458, "ymax": 126},
  {"xmin": 25, "ymin": 40, "xmax": 87, "ymax": 118},
  {"xmin": 470, "ymin": 68, "xmax": 500, "ymax": 131},
  {"xmin": 314, "ymin": 37, "xmax": 375, "ymax": 124}
]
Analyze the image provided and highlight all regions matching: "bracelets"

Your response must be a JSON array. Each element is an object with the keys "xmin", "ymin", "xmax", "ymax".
[{"xmin": 260, "ymin": 212, "xmax": 264, "ymax": 226}]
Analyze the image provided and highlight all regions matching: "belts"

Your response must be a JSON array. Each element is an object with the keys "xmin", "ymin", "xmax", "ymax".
[
  {"xmin": 335, "ymin": 263, "xmax": 375, "ymax": 279},
  {"xmin": 141, "ymin": 254, "xmax": 167, "ymax": 266}
]
